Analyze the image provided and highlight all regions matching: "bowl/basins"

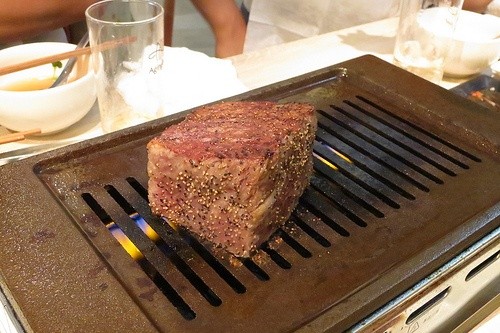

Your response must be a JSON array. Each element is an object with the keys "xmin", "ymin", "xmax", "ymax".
[
  {"xmin": 0, "ymin": 42, "xmax": 99, "ymax": 137},
  {"xmin": 414, "ymin": 7, "xmax": 500, "ymax": 80}
]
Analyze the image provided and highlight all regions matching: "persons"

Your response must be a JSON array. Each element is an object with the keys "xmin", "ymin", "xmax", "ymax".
[
  {"xmin": 0, "ymin": 0, "xmax": 247, "ymax": 59},
  {"xmin": 437, "ymin": 0, "xmax": 493, "ymax": 13}
]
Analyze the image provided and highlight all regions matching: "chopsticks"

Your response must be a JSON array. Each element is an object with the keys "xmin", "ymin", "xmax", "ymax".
[
  {"xmin": 1, "ymin": 35, "xmax": 137, "ymax": 77},
  {"xmin": 0, "ymin": 128, "xmax": 41, "ymax": 143}
]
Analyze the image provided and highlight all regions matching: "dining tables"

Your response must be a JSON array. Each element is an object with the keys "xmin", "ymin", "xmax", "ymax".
[{"xmin": 0, "ymin": 6, "xmax": 500, "ymax": 333}]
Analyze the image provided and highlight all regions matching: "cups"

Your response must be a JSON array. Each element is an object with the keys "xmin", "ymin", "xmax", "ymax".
[
  {"xmin": 85, "ymin": 0, "xmax": 165, "ymax": 132},
  {"xmin": 389, "ymin": 0, "xmax": 465, "ymax": 85}
]
